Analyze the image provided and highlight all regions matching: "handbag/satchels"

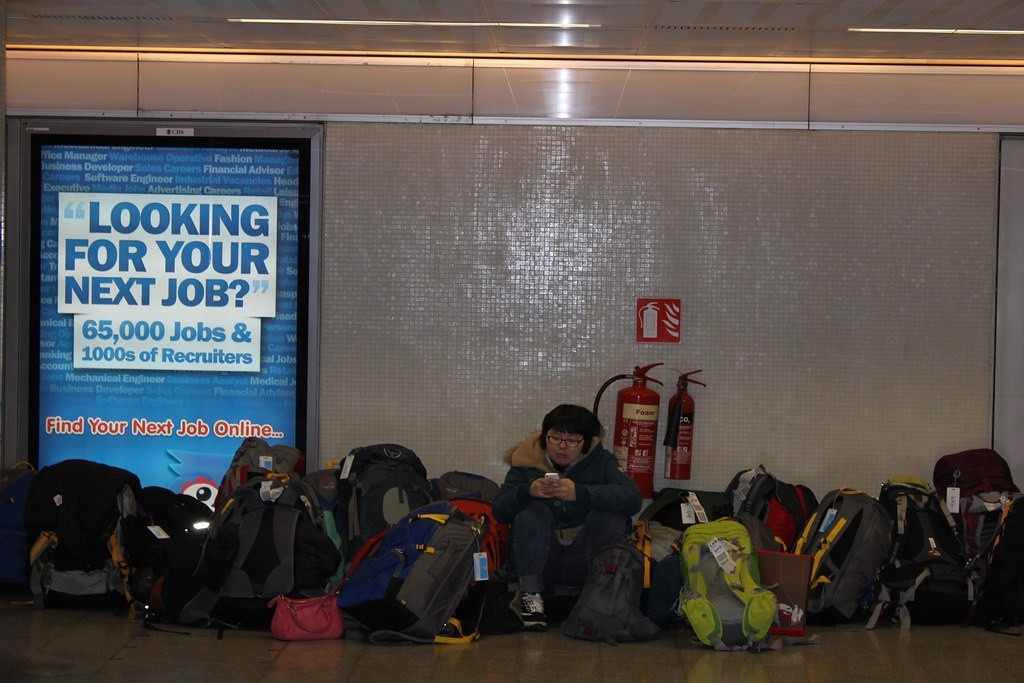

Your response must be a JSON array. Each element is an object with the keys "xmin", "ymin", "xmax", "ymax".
[
  {"xmin": 755, "ymin": 549, "xmax": 808, "ymax": 639},
  {"xmin": 269, "ymin": 595, "xmax": 344, "ymax": 641}
]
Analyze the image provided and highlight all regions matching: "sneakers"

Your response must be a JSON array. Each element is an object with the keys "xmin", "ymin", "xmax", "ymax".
[{"xmin": 520, "ymin": 592, "xmax": 548, "ymax": 633}]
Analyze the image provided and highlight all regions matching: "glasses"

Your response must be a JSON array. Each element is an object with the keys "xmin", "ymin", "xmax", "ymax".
[{"xmin": 544, "ymin": 433, "xmax": 584, "ymax": 449}]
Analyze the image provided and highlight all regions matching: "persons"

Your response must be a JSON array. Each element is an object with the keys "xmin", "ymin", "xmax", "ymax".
[{"xmin": 490, "ymin": 402, "xmax": 639, "ymax": 631}]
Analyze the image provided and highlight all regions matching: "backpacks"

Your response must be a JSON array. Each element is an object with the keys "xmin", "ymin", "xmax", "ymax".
[
  {"xmin": 559, "ymin": 542, "xmax": 660, "ymax": 645},
  {"xmin": 334, "ymin": 442, "xmax": 429, "ymax": 556},
  {"xmin": 790, "ymin": 486, "xmax": 892, "ymax": 628},
  {"xmin": 218, "ymin": 499, "xmax": 317, "ymax": 633},
  {"xmin": 436, "ymin": 469, "xmax": 498, "ymax": 507},
  {"xmin": 0, "ymin": 461, "xmax": 214, "ymax": 635},
  {"xmin": 974, "ymin": 492, "xmax": 1023, "ymax": 636},
  {"xmin": 866, "ymin": 473, "xmax": 963, "ymax": 628},
  {"xmin": 215, "ymin": 437, "xmax": 304, "ymax": 515},
  {"xmin": 302, "ymin": 467, "xmax": 341, "ymax": 514},
  {"xmin": 637, "ymin": 488, "xmax": 714, "ymax": 536},
  {"xmin": 337, "ymin": 502, "xmax": 491, "ymax": 645},
  {"xmin": 676, "ymin": 515, "xmax": 778, "ymax": 651},
  {"xmin": 933, "ymin": 447, "xmax": 1011, "ymax": 568},
  {"xmin": 727, "ymin": 464, "xmax": 819, "ymax": 555},
  {"xmin": 629, "ymin": 518, "xmax": 683, "ymax": 627}
]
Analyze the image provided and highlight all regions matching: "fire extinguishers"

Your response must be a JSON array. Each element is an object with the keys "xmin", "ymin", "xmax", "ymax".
[
  {"xmin": 591, "ymin": 362, "xmax": 666, "ymax": 499},
  {"xmin": 663, "ymin": 370, "xmax": 707, "ymax": 481},
  {"xmin": 638, "ymin": 302, "xmax": 661, "ymax": 339}
]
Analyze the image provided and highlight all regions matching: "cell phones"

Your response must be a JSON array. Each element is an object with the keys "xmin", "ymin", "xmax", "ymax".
[{"xmin": 544, "ymin": 473, "xmax": 559, "ymax": 487}]
